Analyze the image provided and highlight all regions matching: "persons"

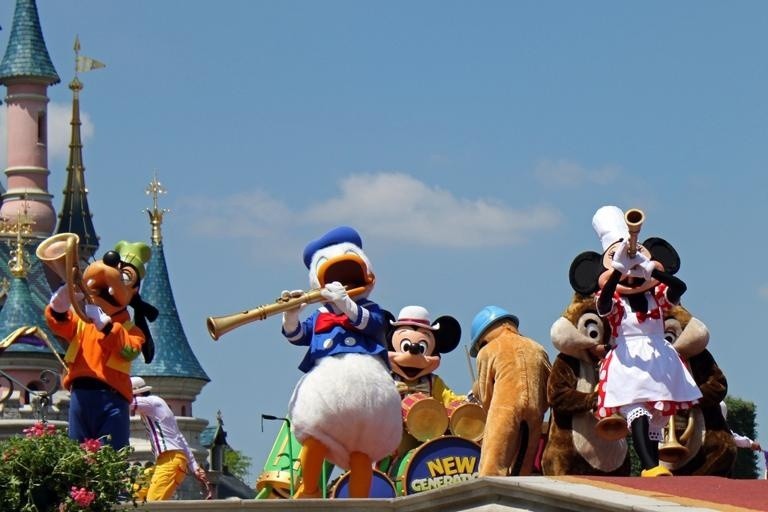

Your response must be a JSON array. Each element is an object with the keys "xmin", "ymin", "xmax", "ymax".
[
  {"xmin": 720, "ymin": 400, "xmax": 762, "ymax": 452},
  {"xmin": 130, "ymin": 376, "xmax": 211, "ymax": 504}
]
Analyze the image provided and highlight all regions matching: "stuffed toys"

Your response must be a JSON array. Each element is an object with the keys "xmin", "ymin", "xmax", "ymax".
[
  {"xmin": 382, "ymin": 304, "xmax": 469, "ymax": 407},
  {"xmin": 276, "ymin": 206, "xmax": 735, "ymax": 499},
  {"xmin": 540, "ymin": 206, "xmax": 729, "ymax": 477},
  {"xmin": 44, "ymin": 241, "xmax": 152, "ymax": 452},
  {"xmin": 277, "ymin": 225, "xmax": 402, "ymax": 496},
  {"xmin": 468, "ymin": 305, "xmax": 551, "ymax": 478}
]
